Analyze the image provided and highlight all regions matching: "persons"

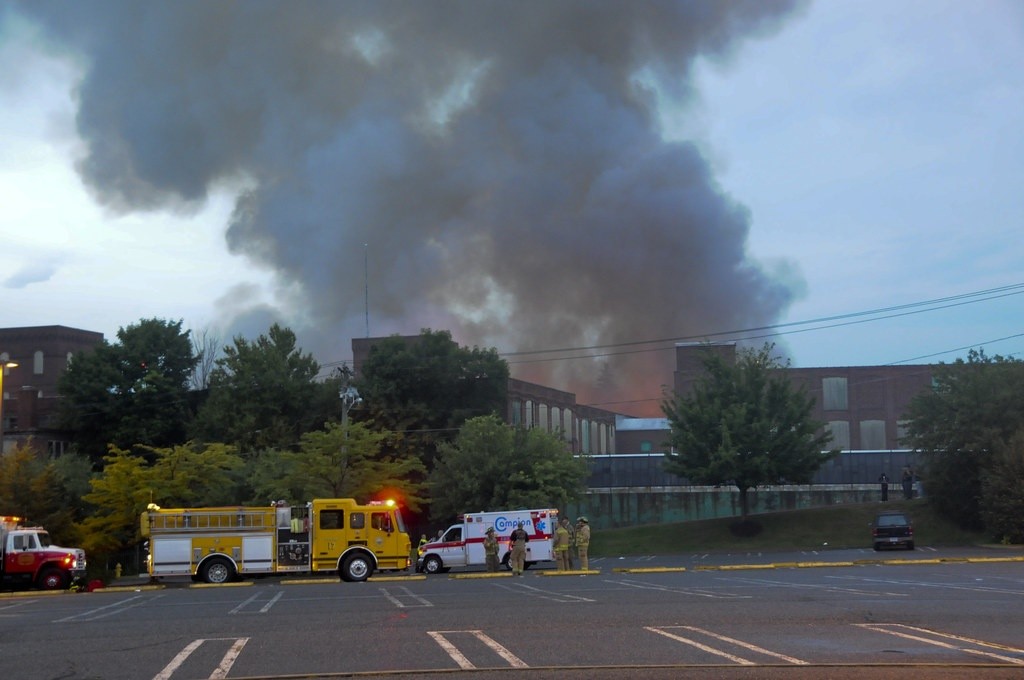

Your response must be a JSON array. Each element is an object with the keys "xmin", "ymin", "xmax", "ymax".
[
  {"xmin": 415, "ymin": 534, "xmax": 428, "ymax": 572},
  {"xmin": 575, "ymin": 517, "xmax": 590, "ymax": 570},
  {"xmin": 552, "ymin": 515, "xmax": 575, "ymax": 570},
  {"xmin": 483, "ymin": 527, "xmax": 500, "ymax": 573},
  {"xmin": 509, "ymin": 523, "xmax": 529, "ymax": 576},
  {"xmin": 438, "ymin": 530, "xmax": 445, "ymax": 542}
]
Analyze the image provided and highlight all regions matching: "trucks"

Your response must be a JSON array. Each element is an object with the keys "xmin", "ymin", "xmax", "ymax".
[{"xmin": 417, "ymin": 508, "xmax": 558, "ymax": 573}]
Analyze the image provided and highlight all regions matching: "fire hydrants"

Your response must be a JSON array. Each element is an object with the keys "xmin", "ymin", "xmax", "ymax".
[{"xmin": 113, "ymin": 562, "xmax": 123, "ymax": 578}]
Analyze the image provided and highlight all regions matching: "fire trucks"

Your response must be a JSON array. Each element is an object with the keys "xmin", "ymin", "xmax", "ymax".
[
  {"xmin": 0, "ymin": 517, "xmax": 87, "ymax": 591},
  {"xmin": 139, "ymin": 498, "xmax": 413, "ymax": 584}
]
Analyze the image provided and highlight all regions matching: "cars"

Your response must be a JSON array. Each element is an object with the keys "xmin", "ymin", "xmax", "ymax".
[{"xmin": 868, "ymin": 510, "xmax": 915, "ymax": 552}]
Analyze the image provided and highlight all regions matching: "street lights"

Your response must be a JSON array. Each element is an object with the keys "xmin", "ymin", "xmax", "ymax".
[{"xmin": 341, "ymin": 398, "xmax": 363, "ymax": 495}]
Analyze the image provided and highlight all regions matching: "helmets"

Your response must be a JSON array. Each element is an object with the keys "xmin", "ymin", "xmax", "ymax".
[
  {"xmin": 485, "ymin": 527, "xmax": 495, "ymax": 535},
  {"xmin": 577, "ymin": 516, "xmax": 588, "ymax": 523}
]
